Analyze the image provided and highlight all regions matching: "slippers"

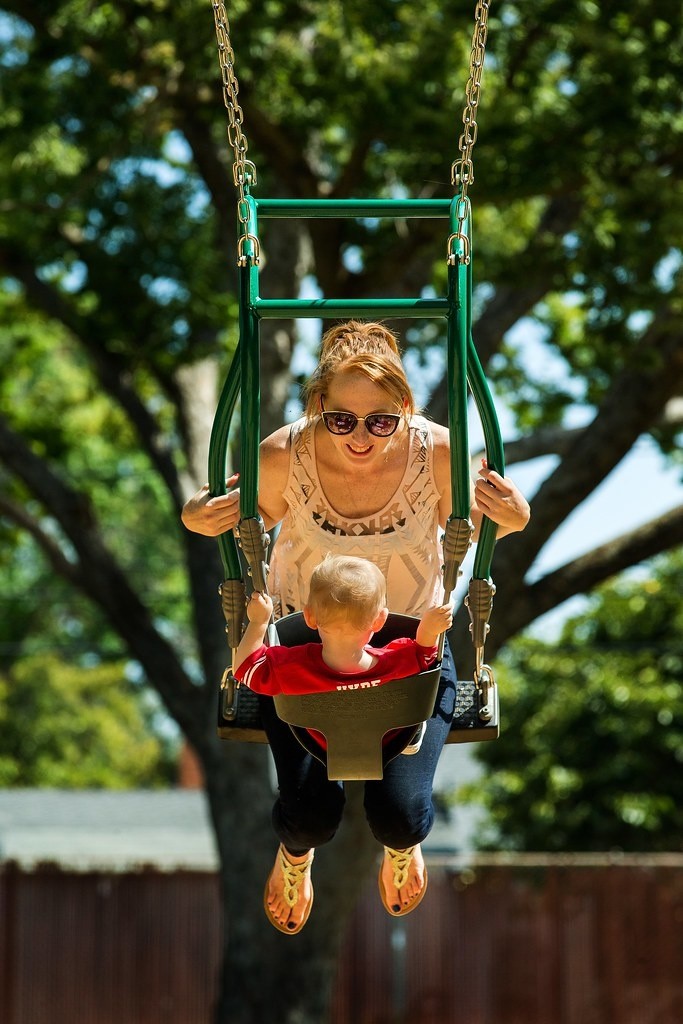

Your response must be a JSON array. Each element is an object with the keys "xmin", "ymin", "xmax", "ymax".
[
  {"xmin": 378, "ymin": 842, "xmax": 427, "ymax": 916},
  {"xmin": 263, "ymin": 842, "xmax": 315, "ymax": 935}
]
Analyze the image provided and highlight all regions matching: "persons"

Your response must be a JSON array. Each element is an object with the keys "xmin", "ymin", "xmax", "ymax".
[
  {"xmin": 182, "ymin": 319, "xmax": 530, "ymax": 935},
  {"xmin": 234, "ymin": 554, "xmax": 452, "ymax": 755}
]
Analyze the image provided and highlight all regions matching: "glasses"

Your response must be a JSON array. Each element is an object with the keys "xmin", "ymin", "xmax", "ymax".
[{"xmin": 320, "ymin": 393, "xmax": 404, "ymax": 437}]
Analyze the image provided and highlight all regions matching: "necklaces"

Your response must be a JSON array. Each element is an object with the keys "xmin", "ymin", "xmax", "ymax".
[{"xmin": 334, "ymin": 445, "xmax": 391, "ymax": 517}]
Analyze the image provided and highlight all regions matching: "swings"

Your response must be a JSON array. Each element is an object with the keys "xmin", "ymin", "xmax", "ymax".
[{"xmin": 206, "ymin": 0, "xmax": 506, "ymax": 781}]
